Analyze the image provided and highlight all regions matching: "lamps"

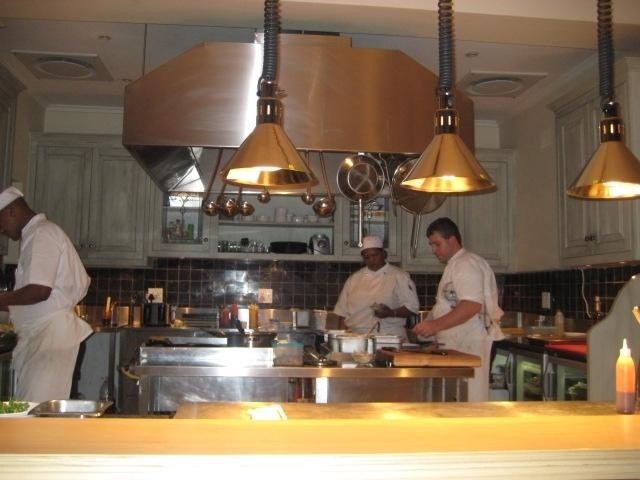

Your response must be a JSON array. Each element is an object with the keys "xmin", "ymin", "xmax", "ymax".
[
  {"xmin": 400, "ymin": 1, "xmax": 498, "ymax": 197},
  {"xmin": 563, "ymin": 1, "xmax": 640, "ymax": 199},
  {"xmin": 217, "ymin": 0, "xmax": 317, "ymax": 190}
]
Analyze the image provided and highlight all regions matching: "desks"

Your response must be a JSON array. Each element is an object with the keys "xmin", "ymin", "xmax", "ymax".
[{"xmin": 173, "ymin": 400, "xmax": 639, "ymax": 426}]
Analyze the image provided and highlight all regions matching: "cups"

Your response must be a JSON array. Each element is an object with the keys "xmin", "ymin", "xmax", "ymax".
[{"xmin": 218, "ymin": 237, "xmax": 269, "ymax": 253}]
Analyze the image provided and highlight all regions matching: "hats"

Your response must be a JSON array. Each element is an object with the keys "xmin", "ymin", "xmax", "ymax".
[
  {"xmin": 360, "ymin": 236, "xmax": 383, "ymax": 253},
  {"xmin": 0, "ymin": 187, "xmax": 24, "ymax": 211}
]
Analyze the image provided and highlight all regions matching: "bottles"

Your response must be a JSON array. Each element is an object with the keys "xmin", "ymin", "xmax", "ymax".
[
  {"xmin": 248, "ymin": 303, "xmax": 260, "ymax": 329},
  {"xmin": 615, "ymin": 338, "xmax": 637, "ymax": 414},
  {"xmin": 555, "ymin": 310, "xmax": 565, "ymax": 338},
  {"xmin": 592, "ymin": 296, "xmax": 605, "ymax": 325}
]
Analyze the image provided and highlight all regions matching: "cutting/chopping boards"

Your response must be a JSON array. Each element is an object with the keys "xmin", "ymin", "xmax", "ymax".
[{"xmin": 375, "ymin": 349, "xmax": 481, "ymax": 368}]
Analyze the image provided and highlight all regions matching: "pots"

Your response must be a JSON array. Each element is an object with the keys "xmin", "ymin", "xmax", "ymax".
[
  {"xmin": 224, "ymin": 315, "xmax": 278, "ymax": 347},
  {"xmin": 144, "ymin": 302, "xmax": 173, "ymax": 327},
  {"xmin": 336, "ymin": 154, "xmax": 386, "ymax": 248}
]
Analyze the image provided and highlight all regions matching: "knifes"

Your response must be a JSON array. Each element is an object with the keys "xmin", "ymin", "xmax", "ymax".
[{"xmin": 406, "ymin": 350, "xmax": 447, "ymax": 356}]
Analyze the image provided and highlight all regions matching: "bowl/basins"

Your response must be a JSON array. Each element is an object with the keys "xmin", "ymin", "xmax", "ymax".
[{"xmin": 232, "ymin": 206, "xmax": 333, "ymax": 224}]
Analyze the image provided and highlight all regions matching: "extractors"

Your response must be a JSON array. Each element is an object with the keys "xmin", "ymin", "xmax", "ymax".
[{"xmin": 120, "ymin": 33, "xmax": 475, "ymax": 198}]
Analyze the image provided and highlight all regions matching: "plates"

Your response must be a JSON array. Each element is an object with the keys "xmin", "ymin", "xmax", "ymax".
[
  {"xmin": 270, "ymin": 241, "xmax": 308, "ymax": 254},
  {"xmin": 310, "ymin": 310, "xmax": 329, "ymax": 330}
]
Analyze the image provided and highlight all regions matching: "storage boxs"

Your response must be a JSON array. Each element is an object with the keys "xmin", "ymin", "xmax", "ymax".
[{"xmin": 272, "ymin": 333, "xmax": 304, "ymax": 366}]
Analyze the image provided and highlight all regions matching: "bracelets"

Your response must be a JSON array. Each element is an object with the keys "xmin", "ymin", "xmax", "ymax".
[{"xmin": 393, "ymin": 310, "xmax": 397, "ymax": 316}]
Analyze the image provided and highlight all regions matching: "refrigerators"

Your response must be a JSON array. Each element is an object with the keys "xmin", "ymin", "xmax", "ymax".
[
  {"xmin": 490, "ymin": 348, "xmax": 512, "ymax": 373},
  {"xmin": 504, "ymin": 346, "xmax": 548, "ymax": 400},
  {"xmin": 541, "ymin": 355, "xmax": 588, "ymax": 400}
]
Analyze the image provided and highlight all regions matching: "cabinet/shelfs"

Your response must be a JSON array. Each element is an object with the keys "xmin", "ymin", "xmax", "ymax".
[
  {"xmin": 1, "ymin": 79, "xmax": 25, "ymax": 255},
  {"xmin": 341, "ymin": 195, "xmax": 399, "ymax": 262},
  {"xmin": 401, "ymin": 150, "xmax": 516, "ymax": 276},
  {"xmin": 213, "ymin": 196, "xmax": 341, "ymax": 262},
  {"xmin": 149, "ymin": 185, "xmax": 212, "ymax": 260},
  {"xmin": 25, "ymin": 130, "xmax": 150, "ymax": 269},
  {"xmin": 557, "ymin": 75, "xmax": 639, "ymax": 271}
]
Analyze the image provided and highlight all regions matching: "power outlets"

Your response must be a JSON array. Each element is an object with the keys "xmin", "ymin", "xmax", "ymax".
[
  {"xmin": 148, "ymin": 288, "xmax": 163, "ymax": 304},
  {"xmin": 257, "ymin": 289, "xmax": 272, "ymax": 304}
]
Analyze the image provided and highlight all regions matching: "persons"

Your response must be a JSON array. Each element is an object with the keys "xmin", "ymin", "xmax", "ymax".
[
  {"xmin": 0, "ymin": 183, "xmax": 94, "ymax": 403},
  {"xmin": 410, "ymin": 217, "xmax": 504, "ymax": 402},
  {"xmin": 331, "ymin": 234, "xmax": 420, "ymax": 344}
]
